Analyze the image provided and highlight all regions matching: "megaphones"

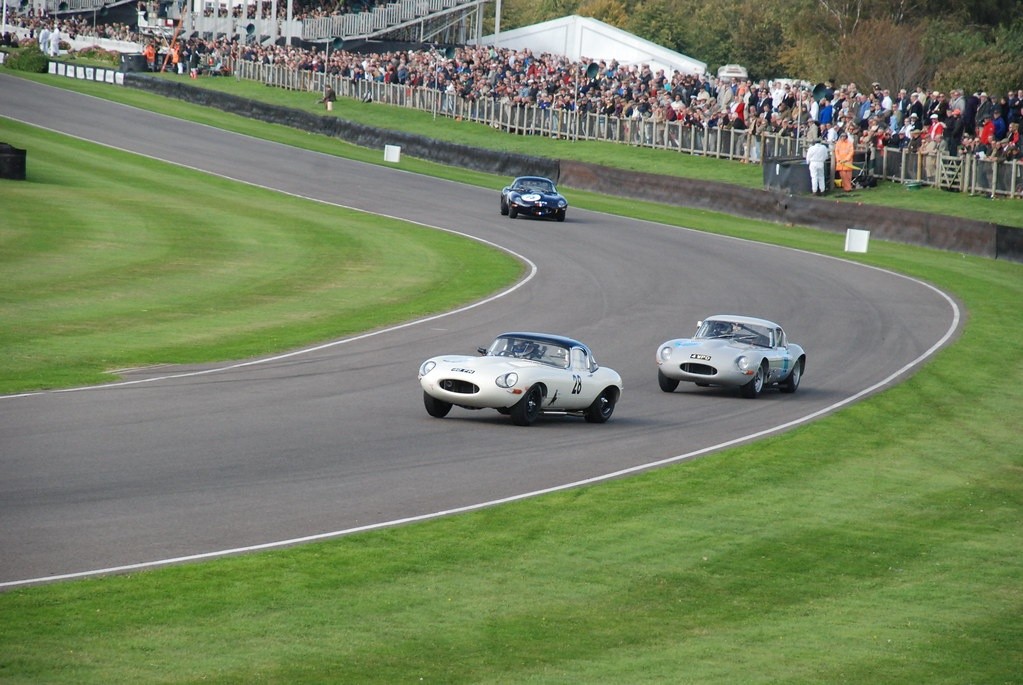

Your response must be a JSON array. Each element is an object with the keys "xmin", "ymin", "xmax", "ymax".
[
  {"xmin": 99, "ymin": 6, "xmax": 109, "ymax": 17},
  {"xmin": 20, "ymin": 0, "xmax": 28, "ymax": 6},
  {"xmin": 241, "ymin": 24, "xmax": 255, "ymax": 34},
  {"xmin": 58, "ymin": 2, "xmax": 69, "ymax": 13},
  {"xmin": 332, "ymin": 37, "xmax": 344, "ymax": 50}
]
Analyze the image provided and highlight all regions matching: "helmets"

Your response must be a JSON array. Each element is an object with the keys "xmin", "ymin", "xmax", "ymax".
[
  {"xmin": 514, "ymin": 339, "xmax": 534, "ymax": 356},
  {"xmin": 714, "ymin": 322, "xmax": 733, "ymax": 335}
]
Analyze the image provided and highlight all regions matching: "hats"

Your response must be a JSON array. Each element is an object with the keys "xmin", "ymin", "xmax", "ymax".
[
  {"xmin": 910, "ymin": 113, "xmax": 918, "ymax": 119},
  {"xmin": 875, "ymin": 85, "xmax": 882, "ymax": 90},
  {"xmin": 930, "ymin": 114, "xmax": 938, "ymax": 118},
  {"xmin": 980, "ymin": 92, "xmax": 988, "ymax": 97},
  {"xmin": 845, "ymin": 114, "xmax": 853, "ymax": 119},
  {"xmin": 751, "ymin": 85, "xmax": 756, "ymax": 89}
]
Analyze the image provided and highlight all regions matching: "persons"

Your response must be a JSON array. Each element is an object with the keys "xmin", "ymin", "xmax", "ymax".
[
  {"xmin": 323, "ymin": 85, "xmax": 336, "ymax": 111},
  {"xmin": 806, "ymin": 139, "xmax": 828, "ymax": 196},
  {"xmin": 0, "ymin": 0, "xmax": 1023, "ymax": 199},
  {"xmin": 714, "ymin": 322, "xmax": 730, "ymax": 335},
  {"xmin": 513, "ymin": 340, "xmax": 535, "ymax": 359},
  {"xmin": 520, "ymin": 181, "xmax": 528, "ymax": 189},
  {"xmin": 834, "ymin": 133, "xmax": 854, "ymax": 191}
]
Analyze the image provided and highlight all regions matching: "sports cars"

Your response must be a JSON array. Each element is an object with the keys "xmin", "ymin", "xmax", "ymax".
[
  {"xmin": 418, "ymin": 331, "xmax": 623, "ymax": 427},
  {"xmin": 499, "ymin": 175, "xmax": 567, "ymax": 221},
  {"xmin": 654, "ymin": 314, "xmax": 808, "ymax": 398}
]
